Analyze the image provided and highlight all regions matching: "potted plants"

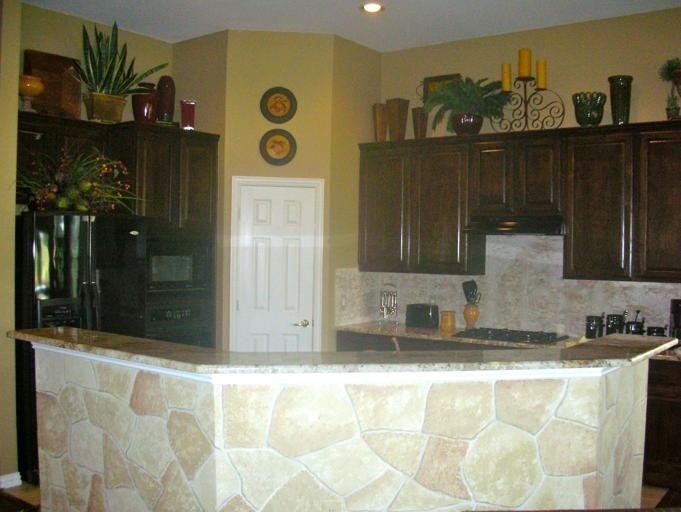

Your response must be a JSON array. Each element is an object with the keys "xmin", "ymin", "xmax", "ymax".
[
  {"xmin": 71, "ymin": 20, "xmax": 167, "ymax": 124},
  {"xmin": 422, "ymin": 77, "xmax": 512, "ymax": 137},
  {"xmin": 658, "ymin": 55, "xmax": 681, "ymax": 124}
]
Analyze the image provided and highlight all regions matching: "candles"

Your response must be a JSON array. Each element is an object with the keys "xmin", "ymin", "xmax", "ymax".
[{"xmin": 501, "ymin": 49, "xmax": 548, "ymax": 92}]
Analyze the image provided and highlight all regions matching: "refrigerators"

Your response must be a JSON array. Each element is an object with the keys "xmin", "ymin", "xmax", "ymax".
[{"xmin": 22, "ymin": 212, "xmax": 151, "ymax": 483}]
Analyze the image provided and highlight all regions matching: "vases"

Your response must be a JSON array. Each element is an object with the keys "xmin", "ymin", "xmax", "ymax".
[
  {"xmin": 372, "ymin": 98, "xmax": 427, "ymax": 143},
  {"xmin": 133, "ymin": 76, "xmax": 174, "ymax": 125},
  {"xmin": 18, "ymin": 76, "xmax": 45, "ymax": 113},
  {"xmin": 572, "ymin": 75, "xmax": 633, "ymax": 128}
]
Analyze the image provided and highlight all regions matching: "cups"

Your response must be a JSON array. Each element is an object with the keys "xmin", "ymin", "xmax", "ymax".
[{"xmin": 440, "ymin": 311, "xmax": 455, "ymax": 331}]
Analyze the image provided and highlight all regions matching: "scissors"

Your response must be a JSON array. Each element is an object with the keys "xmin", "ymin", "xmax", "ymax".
[{"xmin": 469, "ymin": 291, "xmax": 481, "ymax": 305}]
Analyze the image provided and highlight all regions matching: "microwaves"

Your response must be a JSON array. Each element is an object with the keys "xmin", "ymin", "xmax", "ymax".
[{"xmin": 148, "ymin": 244, "xmax": 209, "ymax": 288}]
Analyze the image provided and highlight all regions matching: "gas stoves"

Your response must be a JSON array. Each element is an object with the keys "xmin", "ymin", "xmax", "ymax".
[{"xmin": 453, "ymin": 326, "xmax": 570, "ymax": 347}]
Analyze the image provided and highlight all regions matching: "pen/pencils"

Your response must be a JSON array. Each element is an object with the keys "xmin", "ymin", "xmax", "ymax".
[{"xmin": 602, "ymin": 312, "xmax": 605, "ymax": 318}]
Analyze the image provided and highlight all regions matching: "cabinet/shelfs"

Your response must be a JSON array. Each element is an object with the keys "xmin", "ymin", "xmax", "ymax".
[
  {"xmin": 358, "ymin": 145, "xmax": 486, "ymax": 274},
  {"xmin": 563, "ymin": 130, "xmax": 680, "ymax": 281},
  {"xmin": 114, "ymin": 130, "xmax": 219, "ymax": 238},
  {"xmin": 18, "ymin": 120, "xmax": 112, "ymax": 204},
  {"xmin": 642, "ymin": 364, "xmax": 679, "ymax": 488},
  {"xmin": 478, "ymin": 138, "xmax": 564, "ymax": 234}
]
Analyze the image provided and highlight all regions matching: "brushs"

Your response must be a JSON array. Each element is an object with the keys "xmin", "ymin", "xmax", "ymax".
[{"xmin": 636, "ymin": 310, "xmax": 641, "ymax": 322}]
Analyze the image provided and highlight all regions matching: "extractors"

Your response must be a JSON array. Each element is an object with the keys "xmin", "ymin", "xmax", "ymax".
[{"xmin": 464, "ymin": 216, "xmax": 566, "ymax": 237}]
